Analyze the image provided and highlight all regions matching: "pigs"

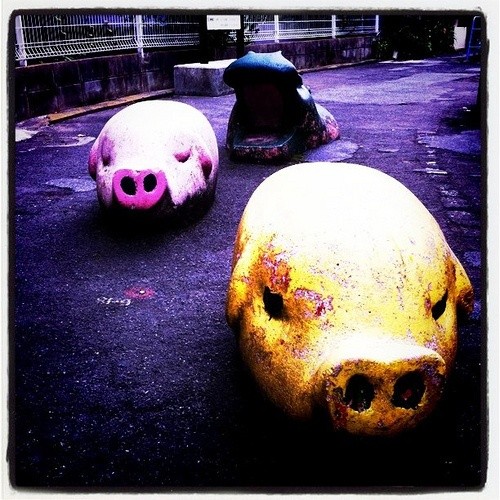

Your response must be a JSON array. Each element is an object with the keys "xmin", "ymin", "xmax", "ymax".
[
  {"xmin": 225, "ymin": 161, "xmax": 475, "ymax": 439},
  {"xmin": 87, "ymin": 99, "xmax": 220, "ymax": 228}
]
